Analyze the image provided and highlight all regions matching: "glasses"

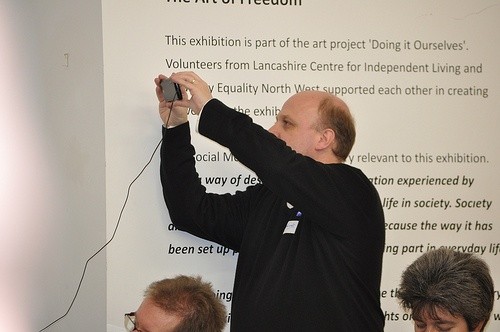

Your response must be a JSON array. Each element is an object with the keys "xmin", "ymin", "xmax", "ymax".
[{"xmin": 124, "ymin": 312, "xmax": 135, "ymax": 332}]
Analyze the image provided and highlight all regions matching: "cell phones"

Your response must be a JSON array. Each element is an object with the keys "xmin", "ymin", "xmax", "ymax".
[{"xmin": 160, "ymin": 79, "xmax": 182, "ymax": 102}]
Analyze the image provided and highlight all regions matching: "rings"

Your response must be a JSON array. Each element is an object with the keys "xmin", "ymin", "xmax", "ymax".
[
  {"xmin": 191, "ymin": 80, "xmax": 196, "ymax": 84},
  {"xmin": 192, "ymin": 73, "xmax": 195, "ymax": 77}
]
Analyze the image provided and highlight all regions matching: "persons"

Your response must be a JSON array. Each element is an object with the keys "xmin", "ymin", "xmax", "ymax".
[
  {"xmin": 396, "ymin": 246, "xmax": 497, "ymax": 332},
  {"xmin": 151, "ymin": 73, "xmax": 387, "ymax": 332},
  {"xmin": 128, "ymin": 274, "xmax": 227, "ymax": 331}
]
